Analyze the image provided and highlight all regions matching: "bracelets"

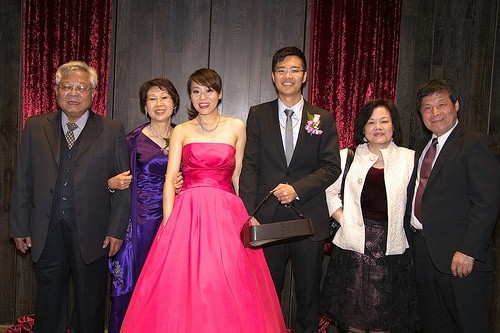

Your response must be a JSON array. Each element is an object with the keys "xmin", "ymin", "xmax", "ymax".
[{"xmin": 107, "ymin": 186, "xmax": 116, "ymax": 194}]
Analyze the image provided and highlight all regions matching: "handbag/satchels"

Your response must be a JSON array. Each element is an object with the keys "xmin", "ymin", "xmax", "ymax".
[{"xmin": 324, "ymin": 213, "xmax": 341, "ymax": 255}]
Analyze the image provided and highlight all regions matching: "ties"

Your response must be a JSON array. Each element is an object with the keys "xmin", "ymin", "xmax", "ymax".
[
  {"xmin": 414, "ymin": 136, "xmax": 440, "ymax": 225},
  {"xmin": 284, "ymin": 108, "xmax": 294, "ymax": 164},
  {"xmin": 66, "ymin": 122, "xmax": 78, "ymax": 148}
]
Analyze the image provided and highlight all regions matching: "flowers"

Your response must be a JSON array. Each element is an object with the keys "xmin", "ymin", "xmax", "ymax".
[
  {"xmin": 163, "ymin": 138, "xmax": 169, "ymax": 151},
  {"xmin": 305, "ymin": 110, "xmax": 325, "ymax": 135}
]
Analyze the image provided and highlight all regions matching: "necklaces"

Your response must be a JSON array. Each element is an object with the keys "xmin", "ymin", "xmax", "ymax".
[
  {"xmin": 195, "ymin": 114, "xmax": 221, "ymax": 132},
  {"xmin": 374, "ymin": 159, "xmax": 383, "ymax": 164},
  {"xmin": 148, "ymin": 124, "xmax": 171, "ymax": 142},
  {"xmin": 277, "ymin": 118, "xmax": 299, "ymax": 130}
]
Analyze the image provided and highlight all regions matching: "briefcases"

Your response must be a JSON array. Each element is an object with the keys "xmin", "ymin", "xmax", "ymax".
[{"xmin": 244, "ymin": 216, "xmax": 315, "ymax": 248}]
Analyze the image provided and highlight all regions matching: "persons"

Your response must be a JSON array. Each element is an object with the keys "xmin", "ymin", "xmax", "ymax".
[
  {"xmin": 318, "ymin": 97, "xmax": 420, "ymax": 333},
  {"xmin": 402, "ymin": 78, "xmax": 500, "ymax": 333},
  {"xmin": 106, "ymin": 77, "xmax": 185, "ymax": 333},
  {"xmin": 237, "ymin": 45, "xmax": 342, "ymax": 333},
  {"xmin": 9, "ymin": 61, "xmax": 135, "ymax": 333},
  {"xmin": 116, "ymin": 67, "xmax": 289, "ymax": 333}
]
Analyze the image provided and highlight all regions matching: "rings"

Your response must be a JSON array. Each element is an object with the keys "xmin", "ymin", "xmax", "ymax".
[{"xmin": 280, "ymin": 197, "xmax": 282, "ymax": 201}]
